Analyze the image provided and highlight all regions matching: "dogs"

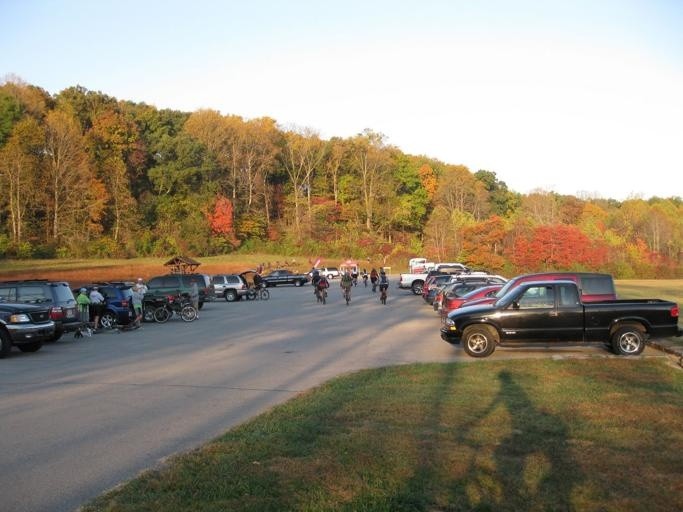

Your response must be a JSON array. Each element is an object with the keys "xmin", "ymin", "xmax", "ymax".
[
  {"xmin": 74, "ymin": 330, "xmax": 83, "ymax": 339},
  {"xmin": 78, "ymin": 328, "xmax": 93, "ymax": 337}
]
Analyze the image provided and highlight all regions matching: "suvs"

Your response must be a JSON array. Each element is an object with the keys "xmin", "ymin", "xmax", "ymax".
[
  {"xmin": 0, "ymin": 302, "xmax": 64, "ymax": 361},
  {"xmin": 0, "ymin": 279, "xmax": 86, "ymax": 344}
]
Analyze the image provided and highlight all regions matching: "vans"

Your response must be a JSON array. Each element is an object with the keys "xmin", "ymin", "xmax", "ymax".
[
  {"xmin": 141, "ymin": 273, "xmax": 208, "ymax": 311},
  {"xmin": 212, "ymin": 269, "xmax": 256, "ymax": 302},
  {"xmin": 457, "ymin": 272, "xmax": 617, "ymax": 309},
  {"xmin": 201, "ymin": 273, "xmax": 218, "ymax": 302}
]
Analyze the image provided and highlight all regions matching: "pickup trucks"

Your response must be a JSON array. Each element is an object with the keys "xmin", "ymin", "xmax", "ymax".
[
  {"xmin": 437, "ymin": 277, "xmax": 681, "ymax": 359},
  {"xmin": 396, "ymin": 263, "xmax": 487, "ymax": 296},
  {"xmin": 109, "ymin": 279, "xmax": 180, "ymax": 324},
  {"xmin": 303, "ymin": 267, "xmax": 339, "ymax": 279},
  {"xmin": 258, "ymin": 269, "xmax": 308, "ymax": 288}
]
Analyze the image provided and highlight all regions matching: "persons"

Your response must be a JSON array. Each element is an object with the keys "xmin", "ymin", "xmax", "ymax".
[
  {"xmin": 241, "ymin": 272, "xmax": 263, "ymax": 300},
  {"xmin": 306, "ymin": 266, "xmax": 389, "ymax": 302},
  {"xmin": 76, "ymin": 277, "xmax": 148, "ymax": 333}
]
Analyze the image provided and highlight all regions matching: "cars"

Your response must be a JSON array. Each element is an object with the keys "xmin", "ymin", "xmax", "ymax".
[
  {"xmin": 70, "ymin": 279, "xmax": 136, "ymax": 331},
  {"xmin": 421, "ymin": 269, "xmax": 508, "ymax": 326}
]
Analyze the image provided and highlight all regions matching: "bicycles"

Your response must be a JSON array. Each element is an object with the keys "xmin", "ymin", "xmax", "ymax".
[
  {"xmin": 152, "ymin": 296, "xmax": 197, "ymax": 322},
  {"xmin": 307, "ymin": 275, "xmax": 390, "ymax": 307},
  {"xmin": 246, "ymin": 282, "xmax": 271, "ymax": 301}
]
